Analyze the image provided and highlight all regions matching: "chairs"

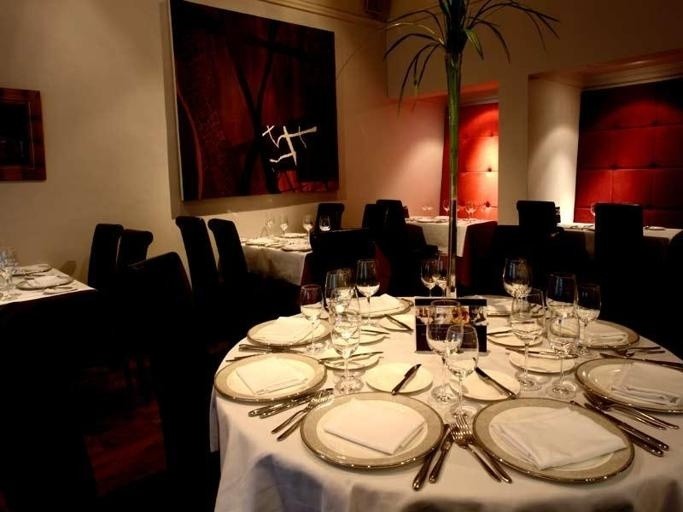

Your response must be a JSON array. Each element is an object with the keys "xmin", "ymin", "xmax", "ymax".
[{"xmin": 0, "ymin": 194, "xmax": 681, "ymax": 511}]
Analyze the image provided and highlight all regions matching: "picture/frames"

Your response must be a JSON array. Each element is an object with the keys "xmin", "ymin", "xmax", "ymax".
[
  {"xmin": 158, "ymin": 0, "xmax": 348, "ymax": 220},
  {"xmin": 0, "ymin": 87, "xmax": 47, "ymax": 183}
]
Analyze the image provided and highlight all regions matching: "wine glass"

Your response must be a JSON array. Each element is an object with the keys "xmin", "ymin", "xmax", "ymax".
[
  {"xmin": 510, "ymin": 260, "xmax": 533, "ymax": 323},
  {"xmin": 443, "ymin": 326, "xmax": 480, "ymax": 415},
  {"xmin": 545, "ymin": 270, "xmax": 579, "ymax": 345},
  {"xmin": 572, "ymin": 283, "xmax": 601, "ymax": 357},
  {"xmin": 510, "ymin": 288, "xmax": 546, "ymax": 391},
  {"xmin": 244, "ymin": 210, "xmax": 331, "ymax": 253},
  {"xmin": 545, "ymin": 319, "xmax": 578, "ymax": 395},
  {"xmin": 426, "ymin": 300, "xmax": 464, "ymax": 399},
  {"xmin": 420, "ymin": 261, "xmax": 436, "ymax": 297},
  {"xmin": 0, "ymin": 243, "xmax": 72, "ymax": 305},
  {"xmin": 354, "ymin": 258, "xmax": 381, "ymax": 326},
  {"xmin": 329, "ymin": 311, "xmax": 364, "ymax": 393},
  {"xmin": 502, "ymin": 263, "xmax": 528, "ymax": 325},
  {"xmin": 414, "ymin": 200, "xmax": 494, "ymax": 227},
  {"xmin": 431, "ymin": 256, "xmax": 449, "ymax": 298},
  {"xmin": 299, "ymin": 285, "xmax": 324, "ymax": 351}
]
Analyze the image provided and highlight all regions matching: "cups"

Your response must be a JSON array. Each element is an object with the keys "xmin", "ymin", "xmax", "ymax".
[
  {"xmin": 323, "ymin": 268, "xmax": 355, "ymax": 314},
  {"xmin": 590, "ymin": 202, "xmax": 595, "ymax": 216},
  {"xmin": 329, "ymin": 287, "xmax": 361, "ymax": 317}
]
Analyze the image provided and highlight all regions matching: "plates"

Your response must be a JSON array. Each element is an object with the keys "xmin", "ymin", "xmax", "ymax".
[
  {"xmin": 488, "ymin": 326, "xmax": 544, "ymax": 348},
  {"xmin": 319, "ymin": 347, "xmax": 379, "ymax": 371},
  {"xmin": 553, "ymin": 317, "xmax": 641, "ymax": 349},
  {"xmin": 364, "ymin": 362, "xmax": 432, "ymax": 393},
  {"xmin": 449, "ymin": 368, "xmax": 521, "ymax": 401},
  {"xmin": 646, "ymin": 225, "xmax": 665, "ymax": 230},
  {"xmin": 213, "ymin": 350, "xmax": 325, "ymax": 404},
  {"xmin": 573, "ymin": 359, "xmax": 682, "ymax": 413},
  {"xmin": 325, "ymin": 324, "xmax": 386, "ymax": 344},
  {"xmin": 350, "ymin": 296, "xmax": 410, "ymax": 316},
  {"xmin": 302, "ymin": 391, "xmax": 442, "ymax": 469},
  {"xmin": 472, "ymin": 396, "xmax": 635, "ymax": 481},
  {"xmin": 378, "ymin": 314, "xmax": 416, "ymax": 332},
  {"xmin": 248, "ymin": 316, "xmax": 331, "ymax": 346},
  {"xmin": 509, "ymin": 346, "xmax": 577, "ymax": 374}
]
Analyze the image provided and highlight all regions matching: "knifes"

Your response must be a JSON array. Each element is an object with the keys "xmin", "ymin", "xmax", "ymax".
[
  {"xmin": 430, "ymin": 428, "xmax": 458, "ymax": 484},
  {"xmin": 391, "ymin": 364, "xmax": 422, "ymax": 395},
  {"xmin": 319, "ymin": 351, "xmax": 384, "ymax": 361},
  {"xmin": 474, "ymin": 366, "xmax": 516, "ymax": 398},
  {"xmin": 570, "ymin": 398, "xmax": 667, "ymax": 457},
  {"xmin": 599, "ymin": 345, "xmax": 683, "ymax": 370},
  {"xmin": 250, "ymin": 388, "xmax": 332, "ymax": 440},
  {"xmin": 409, "ymin": 420, "xmax": 446, "ymax": 490},
  {"xmin": 384, "ymin": 314, "xmax": 413, "ymax": 332}
]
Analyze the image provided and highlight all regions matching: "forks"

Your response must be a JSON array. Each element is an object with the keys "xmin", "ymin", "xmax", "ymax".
[
  {"xmin": 453, "ymin": 414, "xmax": 513, "ymax": 482},
  {"xmin": 447, "ymin": 421, "xmax": 502, "ymax": 481}
]
